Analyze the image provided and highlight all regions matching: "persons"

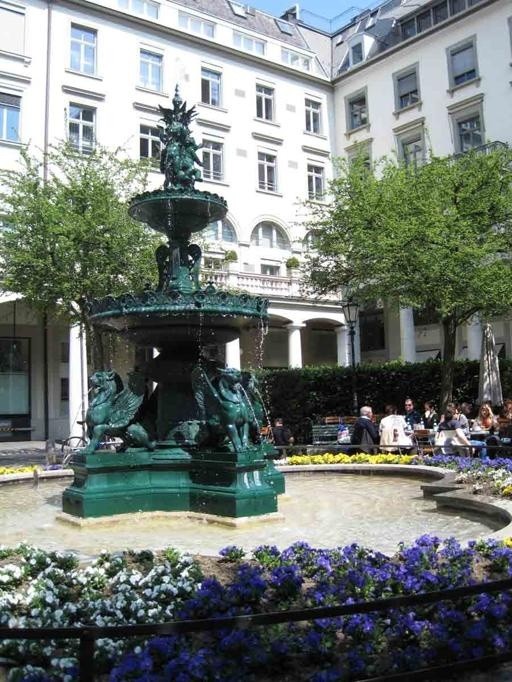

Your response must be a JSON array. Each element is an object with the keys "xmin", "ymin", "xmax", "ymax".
[
  {"xmin": 269, "ymin": 418, "xmax": 295, "ymax": 457},
  {"xmin": 351, "ymin": 397, "xmax": 511, "ymax": 461}
]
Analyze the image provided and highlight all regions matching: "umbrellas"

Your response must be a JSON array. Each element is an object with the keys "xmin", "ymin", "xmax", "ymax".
[{"xmin": 478, "ymin": 324, "xmax": 504, "ymax": 407}]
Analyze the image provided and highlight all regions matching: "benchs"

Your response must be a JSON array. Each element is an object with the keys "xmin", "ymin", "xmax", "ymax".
[{"xmin": 309, "ymin": 413, "xmax": 512, "ymax": 458}]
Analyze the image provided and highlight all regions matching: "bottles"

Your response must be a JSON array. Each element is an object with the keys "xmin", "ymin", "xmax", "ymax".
[{"xmin": 405, "ymin": 416, "xmax": 438, "ymax": 434}]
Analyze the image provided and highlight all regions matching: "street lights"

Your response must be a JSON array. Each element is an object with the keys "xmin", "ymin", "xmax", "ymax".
[{"xmin": 340, "ymin": 296, "xmax": 361, "ymax": 414}]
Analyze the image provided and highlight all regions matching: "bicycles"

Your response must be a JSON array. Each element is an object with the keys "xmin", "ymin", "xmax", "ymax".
[{"xmin": 61, "ymin": 420, "xmax": 116, "ymax": 454}]
